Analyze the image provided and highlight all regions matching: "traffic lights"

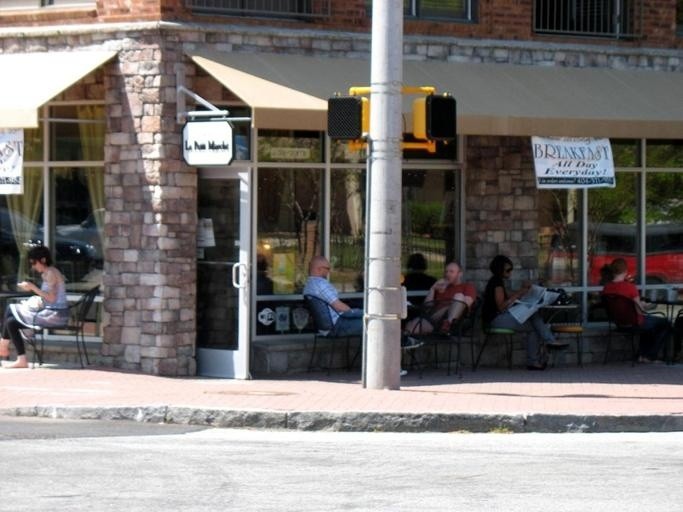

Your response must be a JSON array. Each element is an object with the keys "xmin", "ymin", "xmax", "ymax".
[
  {"xmin": 413, "ymin": 93, "xmax": 457, "ymax": 144},
  {"xmin": 326, "ymin": 94, "xmax": 367, "ymax": 142}
]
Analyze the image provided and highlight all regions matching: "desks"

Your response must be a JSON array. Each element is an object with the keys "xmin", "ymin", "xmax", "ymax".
[{"xmin": 0, "ymin": 291, "xmax": 41, "ymax": 367}]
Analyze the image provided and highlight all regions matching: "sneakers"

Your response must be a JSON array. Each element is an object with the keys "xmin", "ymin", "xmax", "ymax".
[
  {"xmin": 400, "ymin": 368, "xmax": 407, "ymax": 376},
  {"xmin": 439, "ymin": 319, "xmax": 452, "ymax": 336},
  {"xmin": 401, "ymin": 335, "xmax": 424, "ymax": 349},
  {"xmin": 547, "ymin": 343, "xmax": 570, "ymax": 349},
  {"xmin": 527, "ymin": 361, "xmax": 548, "ymax": 371}
]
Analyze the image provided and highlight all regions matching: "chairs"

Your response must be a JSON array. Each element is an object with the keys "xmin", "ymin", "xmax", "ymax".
[
  {"xmin": 412, "ymin": 297, "xmax": 468, "ymax": 376},
  {"xmin": 672, "ymin": 309, "xmax": 683, "ymax": 360},
  {"xmin": 603, "ymin": 293, "xmax": 667, "ymax": 365},
  {"xmin": 482, "ymin": 326, "xmax": 515, "ymax": 368},
  {"xmin": 304, "ymin": 293, "xmax": 363, "ymax": 375},
  {"xmin": 548, "ymin": 302, "xmax": 584, "ymax": 365},
  {"xmin": 32, "ymin": 283, "xmax": 101, "ymax": 367},
  {"xmin": 438, "ymin": 295, "xmax": 480, "ymax": 369}
]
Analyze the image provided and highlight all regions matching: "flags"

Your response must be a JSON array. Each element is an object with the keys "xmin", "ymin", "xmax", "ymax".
[{"xmin": 531, "ymin": 134, "xmax": 619, "ymax": 191}]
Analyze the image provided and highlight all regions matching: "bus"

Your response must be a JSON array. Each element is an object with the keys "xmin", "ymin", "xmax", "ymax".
[{"xmin": 544, "ymin": 219, "xmax": 682, "ymax": 310}]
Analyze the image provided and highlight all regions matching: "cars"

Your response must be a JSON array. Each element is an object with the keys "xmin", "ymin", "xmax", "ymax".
[
  {"xmin": 0, "ymin": 205, "xmax": 97, "ymax": 282},
  {"xmin": 41, "ymin": 206, "xmax": 107, "ymax": 266}
]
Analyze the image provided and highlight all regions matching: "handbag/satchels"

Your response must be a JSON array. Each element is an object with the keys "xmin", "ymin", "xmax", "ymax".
[
  {"xmin": 24, "ymin": 295, "xmax": 46, "ymax": 312},
  {"xmin": 543, "ymin": 289, "xmax": 575, "ymax": 324}
]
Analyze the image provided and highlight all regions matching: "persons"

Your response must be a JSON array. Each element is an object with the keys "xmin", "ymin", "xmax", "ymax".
[
  {"xmin": 404, "ymin": 253, "xmax": 436, "ymax": 290},
  {"xmin": 404, "ymin": 259, "xmax": 477, "ymax": 332},
  {"xmin": 480, "ymin": 254, "xmax": 569, "ymax": 372},
  {"xmin": 0, "ymin": 245, "xmax": 73, "ymax": 368},
  {"xmin": 302, "ymin": 254, "xmax": 423, "ymax": 380},
  {"xmin": 256, "ymin": 254, "xmax": 276, "ymax": 335},
  {"xmin": 401, "ymin": 253, "xmax": 438, "ymax": 292},
  {"xmin": 603, "ymin": 258, "xmax": 655, "ymax": 366}
]
